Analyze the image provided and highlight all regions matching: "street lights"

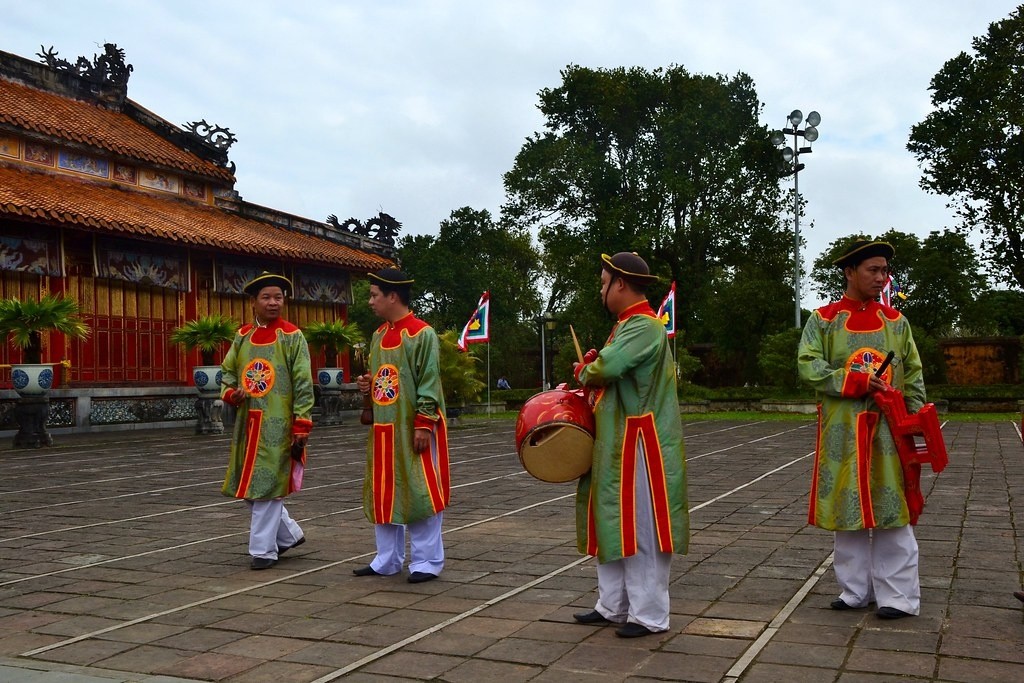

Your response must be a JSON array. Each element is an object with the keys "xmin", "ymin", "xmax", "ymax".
[{"xmin": 770, "ymin": 109, "xmax": 822, "ymax": 329}]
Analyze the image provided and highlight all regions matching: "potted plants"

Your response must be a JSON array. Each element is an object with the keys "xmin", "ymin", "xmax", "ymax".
[
  {"xmin": 0, "ymin": 292, "xmax": 91, "ymax": 397},
  {"xmin": 168, "ymin": 314, "xmax": 240, "ymax": 393},
  {"xmin": 300, "ymin": 317, "xmax": 365, "ymax": 392}
]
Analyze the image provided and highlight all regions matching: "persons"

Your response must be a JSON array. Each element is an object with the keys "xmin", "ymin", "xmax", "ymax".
[
  {"xmin": 496, "ymin": 375, "xmax": 511, "ymax": 390},
  {"xmin": 220, "ymin": 270, "xmax": 314, "ymax": 570},
  {"xmin": 573, "ymin": 248, "xmax": 690, "ymax": 638},
  {"xmin": 352, "ymin": 265, "xmax": 451, "ymax": 584},
  {"xmin": 798, "ymin": 239, "xmax": 927, "ymax": 620}
]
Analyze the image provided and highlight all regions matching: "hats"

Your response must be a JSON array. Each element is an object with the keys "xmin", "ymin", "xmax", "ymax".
[
  {"xmin": 835, "ymin": 238, "xmax": 895, "ymax": 269},
  {"xmin": 600, "ymin": 252, "xmax": 660, "ymax": 283},
  {"xmin": 244, "ymin": 270, "xmax": 293, "ymax": 298},
  {"xmin": 367, "ymin": 266, "xmax": 414, "ymax": 290}
]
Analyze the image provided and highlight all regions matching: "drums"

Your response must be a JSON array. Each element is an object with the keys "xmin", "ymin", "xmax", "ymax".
[{"xmin": 516, "ymin": 390, "xmax": 595, "ymax": 484}]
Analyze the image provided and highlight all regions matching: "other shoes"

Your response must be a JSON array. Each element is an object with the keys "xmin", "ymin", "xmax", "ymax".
[
  {"xmin": 878, "ymin": 606, "xmax": 910, "ymax": 619},
  {"xmin": 830, "ymin": 599, "xmax": 849, "ymax": 610},
  {"xmin": 277, "ymin": 536, "xmax": 306, "ymax": 555},
  {"xmin": 249, "ymin": 557, "xmax": 274, "ymax": 569},
  {"xmin": 356, "ymin": 565, "xmax": 377, "ymax": 576},
  {"xmin": 615, "ymin": 623, "xmax": 651, "ymax": 638},
  {"xmin": 407, "ymin": 571, "xmax": 437, "ymax": 583},
  {"xmin": 573, "ymin": 610, "xmax": 612, "ymax": 624}
]
